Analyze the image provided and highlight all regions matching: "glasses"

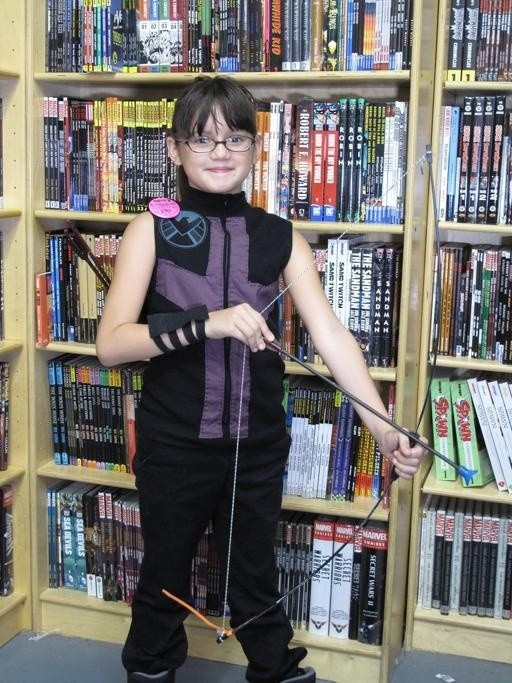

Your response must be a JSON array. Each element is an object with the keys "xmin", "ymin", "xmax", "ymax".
[{"xmin": 175, "ymin": 134, "xmax": 256, "ymax": 153}]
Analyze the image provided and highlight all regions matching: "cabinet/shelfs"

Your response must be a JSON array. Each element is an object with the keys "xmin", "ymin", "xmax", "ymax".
[{"xmin": 1, "ymin": 0, "xmax": 512, "ymax": 683}]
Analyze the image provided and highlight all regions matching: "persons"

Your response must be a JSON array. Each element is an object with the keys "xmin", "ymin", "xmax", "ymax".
[{"xmin": 95, "ymin": 74, "xmax": 429, "ymax": 683}]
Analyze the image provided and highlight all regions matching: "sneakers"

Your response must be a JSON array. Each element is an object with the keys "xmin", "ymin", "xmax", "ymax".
[
  {"xmin": 127, "ymin": 668, "xmax": 175, "ymax": 683},
  {"xmin": 279, "ymin": 666, "xmax": 316, "ymax": 683}
]
